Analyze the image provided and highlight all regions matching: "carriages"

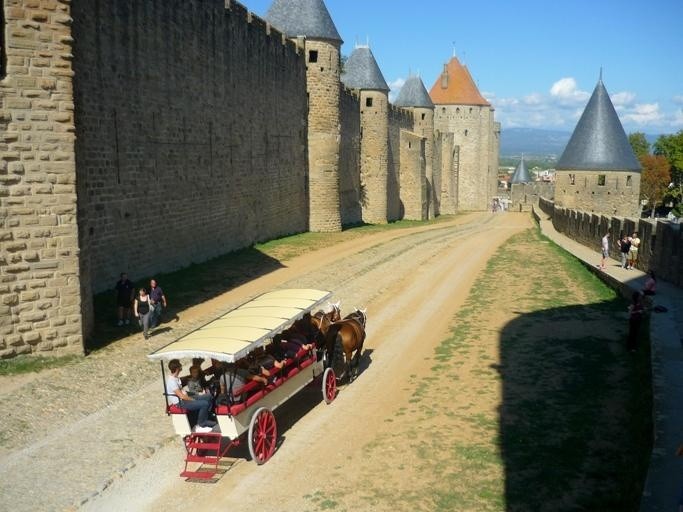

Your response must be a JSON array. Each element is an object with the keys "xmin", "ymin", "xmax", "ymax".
[{"xmin": 147, "ymin": 289, "xmax": 367, "ymax": 478}]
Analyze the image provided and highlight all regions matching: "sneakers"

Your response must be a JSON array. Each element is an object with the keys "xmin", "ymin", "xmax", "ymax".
[
  {"xmin": 627, "ymin": 266, "xmax": 633, "ymax": 270},
  {"xmin": 195, "ymin": 425, "xmax": 212, "ymax": 433},
  {"xmin": 117, "ymin": 320, "xmax": 130, "ymax": 326}
]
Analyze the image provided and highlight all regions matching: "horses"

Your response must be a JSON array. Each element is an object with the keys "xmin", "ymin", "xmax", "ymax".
[
  {"xmin": 310, "ymin": 300, "xmax": 342, "ymax": 336},
  {"xmin": 324, "ymin": 306, "xmax": 368, "ymax": 383}
]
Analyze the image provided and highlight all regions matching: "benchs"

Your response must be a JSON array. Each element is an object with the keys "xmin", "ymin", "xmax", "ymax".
[{"xmin": 168, "ymin": 334, "xmax": 323, "ymax": 436}]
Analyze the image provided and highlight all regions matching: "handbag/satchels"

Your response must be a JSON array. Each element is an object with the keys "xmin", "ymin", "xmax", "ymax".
[{"xmin": 216, "ymin": 393, "xmax": 233, "ymax": 405}]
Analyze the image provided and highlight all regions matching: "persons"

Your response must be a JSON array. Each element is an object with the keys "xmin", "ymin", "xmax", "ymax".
[
  {"xmin": 602, "ymin": 233, "xmax": 611, "ymax": 269},
  {"xmin": 148, "ymin": 279, "xmax": 167, "ymax": 328},
  {"xmin": 166, "ymin": 313, "xmax": 321, "ymax": 432},
  {"xmin": 617, "ymin": 232, "xmax": 640, "ymax": 270},
  {"xmin": 115, "ymin": 272, "xmax": 136, "ymax": 326},
  {"xmin": 135, "ymin": 288, "xmax": 155, "ymax": 340},
  {"xmin": 628, "ymin": 291, "xmax": 647, "ymax": 353},
  {"xmin": 640, "ymin": 269, "xmax": 657, "ymax": 299}
]
